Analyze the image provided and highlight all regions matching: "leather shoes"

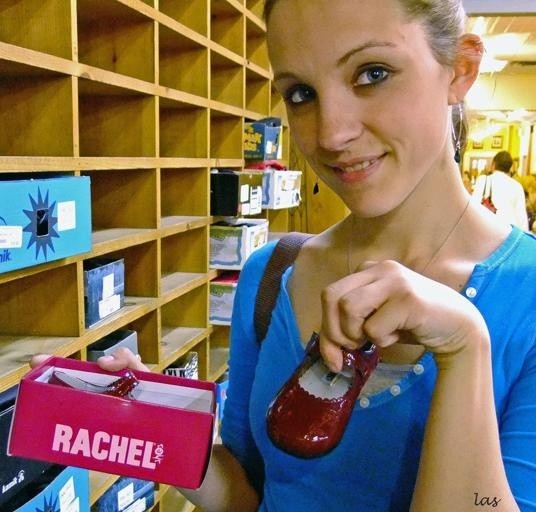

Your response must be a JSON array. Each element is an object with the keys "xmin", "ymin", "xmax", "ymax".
[
  {"xmin": 266, "ymin": 328, "xmax": 382, "ymax": 460},
  {"xmin": 46, "ymin": 369, "xmax": 140, "ymax": 401}
]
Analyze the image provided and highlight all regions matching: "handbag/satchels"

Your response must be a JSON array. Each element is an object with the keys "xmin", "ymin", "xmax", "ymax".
[{"xmin": 481, "ymin": 194, "xmax": 498, "ymax": 214}]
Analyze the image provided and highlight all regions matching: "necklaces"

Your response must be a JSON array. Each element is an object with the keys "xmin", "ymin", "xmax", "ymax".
[{"xmin": 347, "ymin": 195, "xmax": 472, "ymax": 277}]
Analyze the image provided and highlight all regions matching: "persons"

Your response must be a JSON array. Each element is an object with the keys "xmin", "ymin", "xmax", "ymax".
[
  {"xmin": 30, "ymin": 0, "xmax": 535, "ymax": 511},
  {"xmin": 472, "ymin": 151, "xmax": 530, "ymax": 229}
]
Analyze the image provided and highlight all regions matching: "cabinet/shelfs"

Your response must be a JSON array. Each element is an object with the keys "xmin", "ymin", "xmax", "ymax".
[{"xmin": 0, "ymin": 0, "xmax": 299, "ymax": 512}]
